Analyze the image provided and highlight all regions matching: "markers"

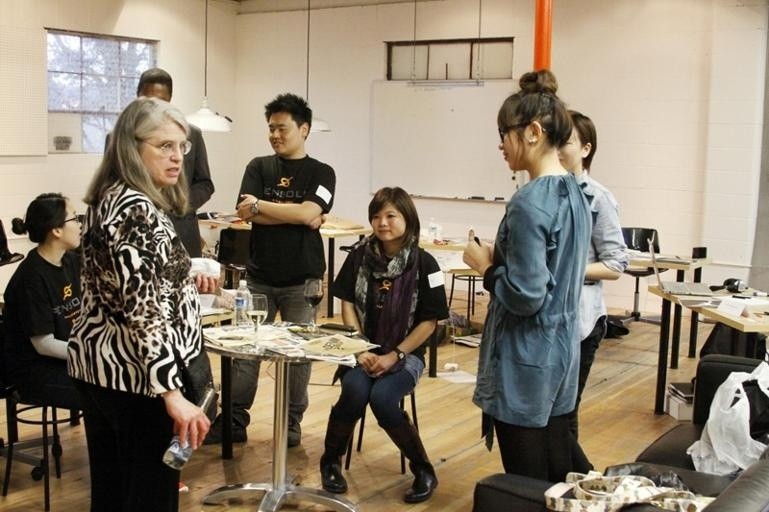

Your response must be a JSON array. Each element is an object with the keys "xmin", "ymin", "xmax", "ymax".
[{"xmin": 469, "ymin": 196, "xmax": 484, "ymax": 199}]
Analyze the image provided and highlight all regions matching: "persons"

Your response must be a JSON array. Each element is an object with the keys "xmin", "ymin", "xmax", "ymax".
[
  {"xmin": 104, "ymin": 65, "xmax": 216, "ymax": 259},
  {"xmin": 318, "ymin": 185, "xmax": 450, "ymax": 507},
  {"xmin": 202, "ymin": 91, "xmax": 337, "ymax": 448},
  {"xmin": 553, "ymin": 107, "xmax": 631, "ymax": 441},
  {"xmin": 66, "ymin": 94, "xmax": 220, "ymax": 512},
  {"xmin": 0, "ymin": 190, "xmax": 85, "ymax": 412},
  {"xmin": 461, "ymin": 68, "xmax": 594, "ymax": 485}
]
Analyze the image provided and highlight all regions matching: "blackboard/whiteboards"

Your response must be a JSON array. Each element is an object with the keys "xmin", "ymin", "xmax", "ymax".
[{"xmin": 370, "ymin": 80, "xmax": 523, "ymax": 204}]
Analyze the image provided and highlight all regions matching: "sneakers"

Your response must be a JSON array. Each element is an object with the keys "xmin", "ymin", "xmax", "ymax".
[
  {"xmin": 202, "ymin": 414, "xmax": 248, "ymax": 444},
  {"xmin": 288, "ymin": 417, "xmax": 301, "ymax": 446}
]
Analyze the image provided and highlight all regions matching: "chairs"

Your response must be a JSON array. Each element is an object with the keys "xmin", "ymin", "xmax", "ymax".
[
  {"xmin": 619, "ymin": 228, "xmax": 668, "ymax": 327},
  {"xmin": 0, "ymin": 300, "xmax": 87, "ymax": 511}
]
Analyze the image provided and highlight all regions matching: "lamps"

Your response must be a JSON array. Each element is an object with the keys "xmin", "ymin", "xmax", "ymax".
[
  {"xmin": 182, "ymin": 0, "xmax": 232, "ymax": 131},
  {"xmin": 292, "ymin": 1, "xmax": 330, "ymax": 134}
]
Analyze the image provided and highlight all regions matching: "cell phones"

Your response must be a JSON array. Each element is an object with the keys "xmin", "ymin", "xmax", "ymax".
[{"xmin": 322, "ymin": 323, "xmax": 355, "ymax": 330}]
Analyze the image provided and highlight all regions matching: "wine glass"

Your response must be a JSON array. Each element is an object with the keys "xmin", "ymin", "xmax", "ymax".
[
  {"xmin": 247, "ymin": 294, "xmax": 269, "ymax": 348},
  {"xmin": 304, "ymin": 279, "xmax": 324, "ymax": 332}
]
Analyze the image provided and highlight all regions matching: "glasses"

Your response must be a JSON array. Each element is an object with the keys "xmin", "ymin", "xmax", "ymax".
[
  {"xmin": 134, "ymin": 138, "xmax": 193, "ymax": 159},
  {"xmin": 498, "ymin": 122, "xmax": 547, "ymax": 143},
  {"xmin": 64, "ymin": 215, "xmax": 79, "ymax": 223}
]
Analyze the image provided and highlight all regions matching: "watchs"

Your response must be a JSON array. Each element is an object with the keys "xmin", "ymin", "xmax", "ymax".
[
  {"xmin": 248, "ymin": 198, "xmax": 260, "ymax": 215},
  {"xmin": 393, "ymin": 347, "xmax": 405, "ymax": 360}
]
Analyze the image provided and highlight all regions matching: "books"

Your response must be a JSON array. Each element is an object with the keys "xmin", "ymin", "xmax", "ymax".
[
  {"xmin": 667, "ymin": 386, "xmax": 693, "ymax": 404},
  {"xmin": 671, "ymin": 381, "xmax": 695, "ymax": 398},
  {"xmin": 202, "ymin": 324, "xmax": 382, "ymax": 369}
]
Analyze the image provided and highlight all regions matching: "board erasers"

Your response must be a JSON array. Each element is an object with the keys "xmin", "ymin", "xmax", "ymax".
[{"xmin": 495, "ymin": 197, "xmax": 504, "ymax": 200}]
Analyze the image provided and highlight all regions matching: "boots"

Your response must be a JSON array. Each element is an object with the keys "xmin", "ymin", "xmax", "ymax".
[
  {"xmin": 320, "ymin": 405, "xmax": 360, "ymax": 493},
  {"xmin": 378, "ymin": 411, "xmax": 438, "ymax": 504}
]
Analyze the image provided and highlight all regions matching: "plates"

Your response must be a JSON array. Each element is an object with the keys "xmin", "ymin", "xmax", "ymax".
[
  {"xmin": 213, "ymin": 333, "xmax": 256, "ymax": 345},
  {"xmin": 197, "ymin": 212, "xmax": 219, "ymax": 219}
]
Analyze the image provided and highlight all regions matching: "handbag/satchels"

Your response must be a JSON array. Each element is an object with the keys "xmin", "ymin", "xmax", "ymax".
[
  {"xmin": 544, "ymin": 462, "xmax": 717, "ymax": 512},
  {"xmin": 183, "ymin": 350, "xmax": 219, "ymax": 423}
]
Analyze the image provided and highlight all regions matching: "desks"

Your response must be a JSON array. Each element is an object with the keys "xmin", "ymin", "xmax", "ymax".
[
  {"xmin": 197, "ymin": 211, "xmax": 371, "ymax": 319},
  {"xmin": 196, "ymin": 325, "xmax": 366, "ymax": 512},
  {"xmin": 625, "ymin": 256, "xmax": 769, "ymax": 417},
  {"xmin": 416, "ymin": 237, "xmax": 487, "ymax": 382}
]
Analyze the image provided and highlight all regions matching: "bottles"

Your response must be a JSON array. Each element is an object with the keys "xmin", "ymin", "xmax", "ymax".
[
  {"xmin": 235, "ymin": 280, "xmax": 252, "ymax": 333},
  {"xmin": 427, "ymin": 218, "xmax": 437, "ymax": 242},
  {"xmin": 162, "ymin": 388, "xmax": 217, "ymax": 470}
]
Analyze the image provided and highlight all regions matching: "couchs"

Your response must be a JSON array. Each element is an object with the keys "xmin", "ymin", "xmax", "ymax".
[{"xmin": 475, "ymin": 354, "xmax": 768, "ymax": 512}]
